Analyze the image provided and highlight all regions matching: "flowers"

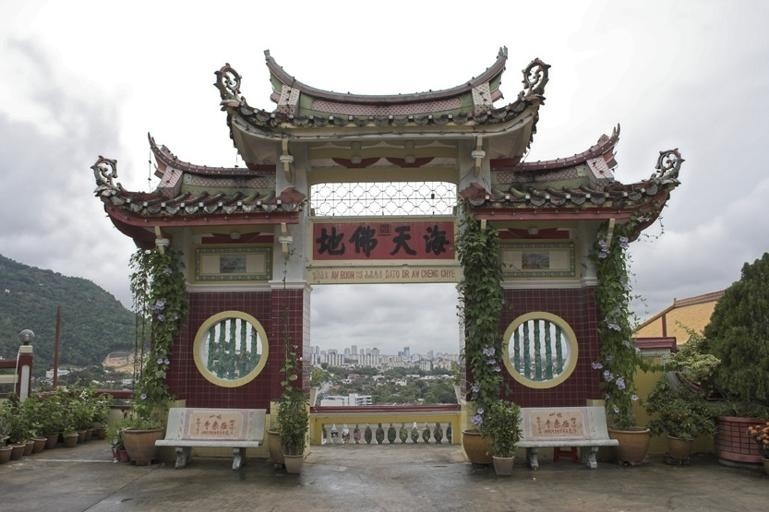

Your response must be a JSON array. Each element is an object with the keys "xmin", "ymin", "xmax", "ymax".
[
  {"xmin": 125, "ymin": 246, "xmax": 187, "ymax": 426},
  {"xmin": 746, "ymin": 422, "xmax": 769, "ymax": 460},
  {"xmin": 454, "ymin": 217, "xmax": 512, "ymax": 426},
  {"xmin": 593, "ymin": 234, "xmax": 648, "ymax": 426}
]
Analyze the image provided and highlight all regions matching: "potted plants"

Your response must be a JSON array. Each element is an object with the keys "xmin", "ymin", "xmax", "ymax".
[
  {"xmin": 0, "ymin": 384, "xmax": 113, "ymax": 464},
  {"xmin": 484, "ymin": 398, "xmax": 520, "ymax": 474},
  {"xmin": 267, "ymin": 306, "xmax": 334, "ymax": 475},
  {"xmin": 650, "ymin": 390, "xmax": 719, "ymax": 465}
]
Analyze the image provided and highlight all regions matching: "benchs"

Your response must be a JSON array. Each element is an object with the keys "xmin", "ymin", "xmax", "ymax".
[
  {"xmin": 155, "ymin": 406, "xmax": 268, "ymax": 473},
  {"xmin": 518, "ymin": 406, "xmax": 618, "ymax": 471}
]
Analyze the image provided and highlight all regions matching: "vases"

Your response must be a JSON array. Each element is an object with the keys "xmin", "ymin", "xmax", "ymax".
[
  {"xmin": 607, "ymin": 426, "xmax": 649, "ymax": 468},
  {"xmin": 763, "ymin": 459, "xmax": 768, "ymax": 474},
  {"xmin": 121, "ymin": 428, "xmax": 164, "ymax": 466},
  {"xmin": 462, "ymin": 428, "xmax": 492, "ymax": 465}
]
